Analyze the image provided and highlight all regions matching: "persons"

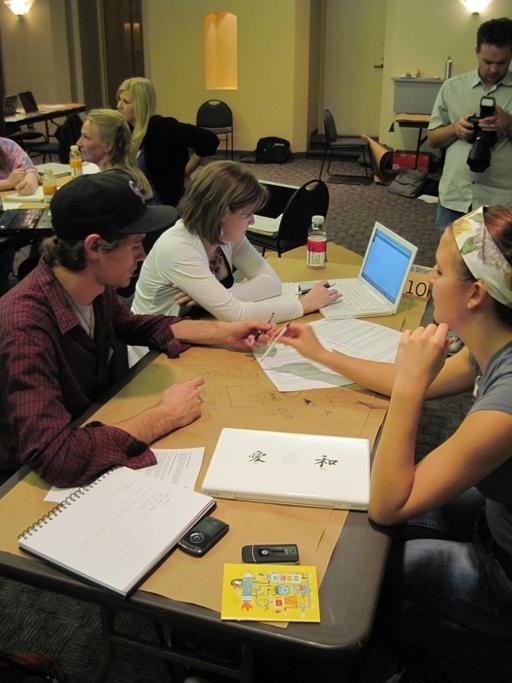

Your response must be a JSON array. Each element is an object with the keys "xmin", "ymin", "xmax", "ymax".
[
  {"xmin": 68, "ymin": 107, "xmax": 164, "ymax": 317},
  {"xmin": 1, "ymin": 168, "xmax": 278, "ymax": 489},
  {"xmin": 0, "ymin": 132, "xmax": 40, "ymax": 196},
  {"xmin": 428, "ymin": 16, "xmax": 511, "ymax": 258},
  {"xmin": 131, "ymin": 160, "xmax": 341, "ymax": 330},
  {"xmin": 116, "ymin": 76, "xmax": 218, "ymax": 298},
  {"xmin": 282, "ymin": 205, "xmax": 512, "ymax": 654}
]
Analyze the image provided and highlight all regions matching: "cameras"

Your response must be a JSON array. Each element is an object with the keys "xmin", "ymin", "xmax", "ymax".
[{"xmin": 458, "ymin": 96, "xmax": 501, "ymax": 175}]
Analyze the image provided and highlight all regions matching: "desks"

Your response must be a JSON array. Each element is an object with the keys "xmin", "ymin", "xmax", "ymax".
[
  {"xmin": 395, "ymin": 114, "xmax": 431, "ymax": 170},
  {"xmin": 4, "ymin": 103, "xmax": 89, "ymax": 127},
  {"xmin": 0, "ymin": 261, "xmax": 439, "ymax": 683}
]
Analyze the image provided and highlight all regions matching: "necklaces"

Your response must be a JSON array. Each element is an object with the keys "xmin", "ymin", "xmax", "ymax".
[{"xmin": 61, "ymin": 284, "xmax": 92, "ymax": 332}]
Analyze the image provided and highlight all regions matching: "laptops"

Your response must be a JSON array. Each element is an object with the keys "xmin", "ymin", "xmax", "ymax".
[
  {"xmin": 5, "ymin": 94, "xmax": 18, "ymax": 116},
  {"xmin": 0, "ymin": 194, "xmax": 53, "ymax": 233},
  {"xmin": 19, "ymin": 92, "xmax": 55, "ymax": 116},
  {"xmin": 297, "ymin": 222, "xmax": 417, "ymax": 323}
]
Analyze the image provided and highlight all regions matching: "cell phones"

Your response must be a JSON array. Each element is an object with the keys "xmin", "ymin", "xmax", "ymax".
[
  {"xmin": 178, "ymin": 515, "xmax": 229, "ymax": 555},
  {"xmin": 242, "ymin": 543, "xmax": 301, "ymax": 564}
]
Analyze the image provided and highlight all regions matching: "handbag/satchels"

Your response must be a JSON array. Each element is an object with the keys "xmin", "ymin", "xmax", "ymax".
[
  {"xmin": 253, "ymin": 136, "xmax": 289, "ymax": 164},
  {"xmin": 388, "ymin": 169, "xmax": 427, "ymax": 199}
]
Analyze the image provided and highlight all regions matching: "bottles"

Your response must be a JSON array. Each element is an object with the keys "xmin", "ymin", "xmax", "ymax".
[
  {"xmin": 306, "ymin": 215, "xmax": 327, "ymax": 270},
  {"xmin": 70, "ymin": 146, "xmax": 83, "ymax": 178},
  {"xmin": 444, "ymin": 55, "xmax": 453, "ymax": 81},
  {"xmin": 43, "ymin": 169, "xmax": 57, "ymax": 207}
]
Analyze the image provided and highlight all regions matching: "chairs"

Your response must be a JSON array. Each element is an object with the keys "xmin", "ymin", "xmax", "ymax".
[
  {"xmin": 246, "ymin": 179, "xmax": 329, "ymax": 258},
  {"xmin": 0, "ymin": 183, "xmax": 53, "ymax": 281},
  {"xmin": 26, "ymin": 113, "xmax": 83, "ymax": 164},
  {"xmin": 196, "ymin": 100, "xmax": 233, "ymax": 160},
  {"xmin": 319, "ymin": 109, "xmax": 374, "ymax": 185},
  {"xmin": 380, "ymin": 589, "xmax": 512, "ymax": 683}
]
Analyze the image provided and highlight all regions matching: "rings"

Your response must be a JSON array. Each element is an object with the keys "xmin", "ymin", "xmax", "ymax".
[{"xmin": 491, "ymin": 123, "xmax": 494, "ymax": 127}]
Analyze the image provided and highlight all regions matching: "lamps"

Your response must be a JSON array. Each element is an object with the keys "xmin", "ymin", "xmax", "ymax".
[
  {"xmin": 4, "ymin": 0, "xmax": 33, "ymax": 17},
  {"xmin": 461, "ymin": 1, "xmax": 489, "ymax": 17}
]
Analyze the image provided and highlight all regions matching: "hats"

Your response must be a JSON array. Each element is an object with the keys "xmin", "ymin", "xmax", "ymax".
[{"xmin": 50, "ymin": 168, "xmax": 177, "ymax": 238}]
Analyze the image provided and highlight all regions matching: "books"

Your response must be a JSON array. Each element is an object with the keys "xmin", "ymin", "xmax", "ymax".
[
  {"xmin": 18, "ymin": 464, "xmax": 215, "ymax": 607},
  {"xmin": 202, "ymin": 426, "xmax": 373, "ymax": 511}
]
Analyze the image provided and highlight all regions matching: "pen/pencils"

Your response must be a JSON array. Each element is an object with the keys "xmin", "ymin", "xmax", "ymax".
[
  {"xmin": 259, "ymin": 322, "xmax": 290, "ymax": 361},
  {"xmin": 295, "ymin": 281, "xmax": 336, "ymax": 295},
  {"xmin": 252, "ymin": 311, "xmax": 275, "ymax": 344}
]
